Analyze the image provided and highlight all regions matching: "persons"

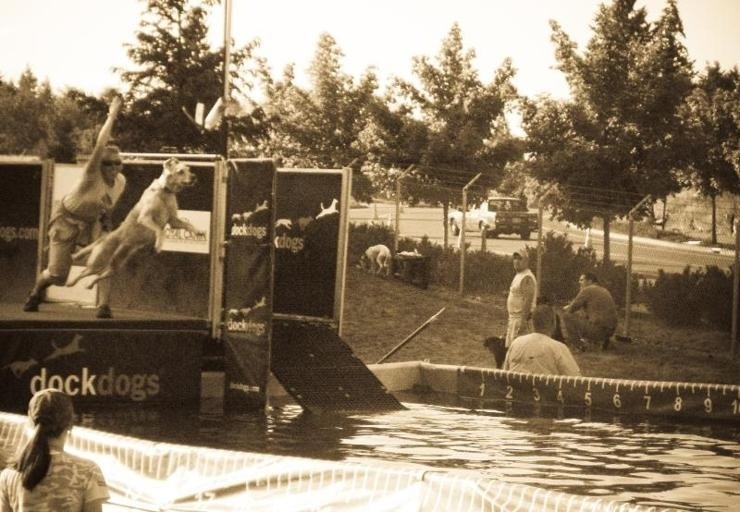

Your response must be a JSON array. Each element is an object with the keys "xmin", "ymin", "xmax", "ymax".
[
  {"xmin": 499, "ymin": 304, "xmax": 580, "ymax": 375},
  {"xmin": 502, "ymin": 249, "xmax": 537, "ymax": 348},
  {"xmin": 21, "ymin": 94, "xmax": 129, "ymax": 319},
  {"xmin": 0, "ymin": 388, "xmax": 110, "ymax": 512},
  {"xmin": 560, "ymin": 272, "xmax": 621, "ymax": 352}
]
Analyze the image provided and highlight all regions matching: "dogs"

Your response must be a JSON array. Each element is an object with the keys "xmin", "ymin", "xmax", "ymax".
[
  {"xmin": 358, "ymin": 243, "xmax": 395, "ymax": 278},
  {"xmin": 484, "ymin": 334, "xmax": 509, "ymax": 369},
  {"xmin": 66, "ymin": 154, "xmax": 207, "ymax": 291}
]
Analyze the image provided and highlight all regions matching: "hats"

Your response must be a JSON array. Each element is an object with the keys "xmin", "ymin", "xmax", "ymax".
[
  {"xmin": 514, "ymin": 249, "xmax": 528, "ymax": 258},
  {"xmin": 28, "ymin": 388, "xmax": 72, "ymax": 426}
]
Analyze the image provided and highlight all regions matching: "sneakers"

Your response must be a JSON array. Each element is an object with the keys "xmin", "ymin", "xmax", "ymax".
[
  {"xmin": 24, "ymin": 290, "xmax": 45, "ymax": 310},
  {"xmin": 96, "ymin": 304, "xmax": 111, "ymax": 318}
]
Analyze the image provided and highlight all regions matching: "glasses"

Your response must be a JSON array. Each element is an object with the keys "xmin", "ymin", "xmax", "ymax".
[{"xmin": 105, "ymin": 159, "xmax": 121, "ymax": 167}]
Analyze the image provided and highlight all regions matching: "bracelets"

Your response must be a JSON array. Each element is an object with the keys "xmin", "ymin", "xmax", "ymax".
[{"xmin": 106, "ymin": 109, "xmax": 120, "ymax": 121}]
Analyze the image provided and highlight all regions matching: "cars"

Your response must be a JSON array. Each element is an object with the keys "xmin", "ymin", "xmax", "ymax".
[{"xmin": 448, "ymin": 196, "xmax": 541, "ymax": 241}]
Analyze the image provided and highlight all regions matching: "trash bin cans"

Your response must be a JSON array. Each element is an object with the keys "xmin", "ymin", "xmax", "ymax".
[{"xmin": 396, "ymin": 254, "xmax": 430, "ymax": 290}]
